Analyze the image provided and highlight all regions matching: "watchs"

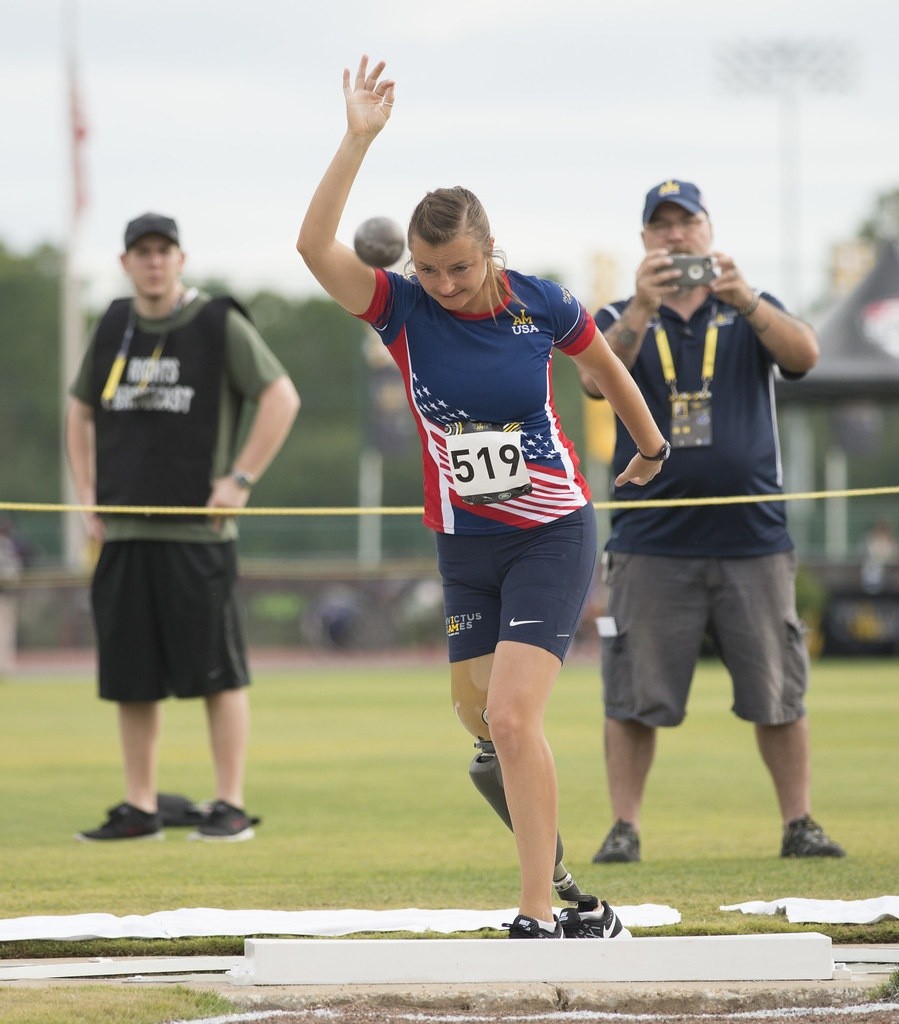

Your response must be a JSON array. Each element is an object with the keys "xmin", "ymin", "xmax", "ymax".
[
  {"xmin": 636, "ymin": 441, "xmax": 671, "ymax": 462},
  {"xmin": 228, "ymin": 468, "xmax": 256, "ymax": 496}
]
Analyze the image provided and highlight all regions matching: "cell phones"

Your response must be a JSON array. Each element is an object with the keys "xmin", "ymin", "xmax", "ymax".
[{"xmin": 655, "ymin": 257, "xmax": 711, "ymax": 286}]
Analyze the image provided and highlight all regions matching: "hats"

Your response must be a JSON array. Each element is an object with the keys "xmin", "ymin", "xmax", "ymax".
[
  {"xmin": 642, "ymin": 179, "xmax": 709, "ymax": 222},
  {"xmin": 124, "ymin": 211, "xmax": 180, "ymax": 249}
]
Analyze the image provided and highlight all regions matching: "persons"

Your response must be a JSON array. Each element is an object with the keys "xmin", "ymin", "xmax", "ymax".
[
  {"xmin": 297, "ymin": 53, "xmax": 673, "ymax": 941},
  {"xmin": 66, "ymin": 210, "xmax": 300, "ymax": 845},
  {"xmin": 581, "ymin": 177, "xmax": 848, "ymax": 865}
]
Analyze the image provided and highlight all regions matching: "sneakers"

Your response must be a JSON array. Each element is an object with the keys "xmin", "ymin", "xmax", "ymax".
[
  {"xmin": 188, "ymin": 799, "xmax": 255, "ymax": 842},
  {"xmin": 780, "ymin": 813, "xmax": 846, "ymax": 858},
  {"xmin": 592, "ymin": 818, "xmax": 641, "ymax": 863},
  {"xmin": 77, "ymin": 802, "xmax": 166, "ymax": 843},
  {"xmin": 501, "ymin": 899, "xmax": 630, "ymax": 938}
]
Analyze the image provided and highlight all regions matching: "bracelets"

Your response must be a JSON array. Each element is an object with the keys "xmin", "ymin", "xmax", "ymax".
[{"xmin": 739, "ymin": 293, "xmax": 760, "ymax": 316}]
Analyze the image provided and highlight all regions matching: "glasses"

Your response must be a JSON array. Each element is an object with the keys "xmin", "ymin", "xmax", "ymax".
[{"xmin": 643, "ymin": 215, "xmax": 707, "ymax": 230}]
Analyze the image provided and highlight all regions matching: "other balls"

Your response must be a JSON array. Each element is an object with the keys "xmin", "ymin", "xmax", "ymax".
[{"xmin": 352, "ymin": 216, "xmax": 406, "ymax": 270}]
